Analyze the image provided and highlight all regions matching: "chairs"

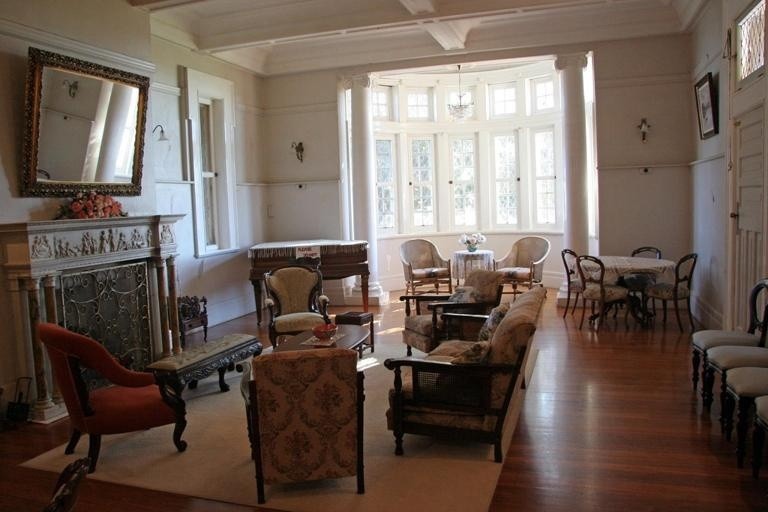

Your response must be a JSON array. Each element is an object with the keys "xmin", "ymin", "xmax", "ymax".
[
  {"xmin": 643, "ymin": 252, "xmax": 698, "ymax": 332},
  {"xmin": 401, "ymin": 239, "xmax": 452, "ymax": 303},
  {"xmin": 563, "ymin": 250, "xmax": 595, "ymax": 317},
  {"xmin": 614, "ymin": 247, "xmax": 660, "ymax": 317},
  {"xmin": 42, "ymin": 454, "xmax": 91, "ymax": 511},
  {"xmin": 726, "ymin": 367, "xmax": 767, "ymax": 467},
  {"xmin": 401, "ymin": 271, "xmax": 503, "ymax": 355},
  {"xmin": 494, "ymin": 235, "xmax": 550, "ymax": 302},
  {"xmin": 577, "ymin": 255, "xmax": 625, "ymax": 334},
  {"xmin": 264, "ymin": 265, "xmax": 331, "ymax": 348},
  {"xmin": 693, "ymin": 277, "xmax": 768, "ymax": 397},
  {"xmin": 168, "ymin": 297, "xmax": 207, "ymax": 347},
  {"xmin": 36, "ymin": 323, "xmax": 188, "ymax": 474},
  {"xmin": 705, "ymin": 346, "xmax": 768, "ymax": 436},
  {"xmin": 753, "ymin": 396, "xmax": 767, "ymax": 479},
  {"xmin": 237, "ymin": 348, "xmax": 364, "ymax": 504}
]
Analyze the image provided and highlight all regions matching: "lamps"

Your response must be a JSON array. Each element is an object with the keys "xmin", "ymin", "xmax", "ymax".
[
  {"xmin": 152, "ymin": 125, "xmax": 168, "ymax": 142},
  {"xmin": 637, "ymin": 118, "xmax": 650, "ymax": 142},
  {"xmin": 62, "ymin": 80, "xmax": 78, "ymax": 97},
  {"xmin": 289, "ymin": 142, "xmax": 303, "ymax": 162},
  {"xmin": 445, "ymin": 65, "xmax": 473, "ymax": 121}
]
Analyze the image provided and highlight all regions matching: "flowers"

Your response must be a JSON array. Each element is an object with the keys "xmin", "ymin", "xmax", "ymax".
[
  {"xmin": 54, "ymin": 192, "xmax": 128, "ymax": 220},
  {"xmin": 459, "ymin": 234, "xmax": 486, "ymax": 247}
]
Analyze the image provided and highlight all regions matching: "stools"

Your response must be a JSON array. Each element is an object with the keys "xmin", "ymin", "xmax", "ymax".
[{"xmin": 336, "ymin": 310, "xmax": 373, "ymax": 358}]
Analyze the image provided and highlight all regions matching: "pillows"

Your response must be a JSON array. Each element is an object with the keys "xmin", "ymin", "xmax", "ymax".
[
  {"xmin": 450, "ymin": 286, "xmax": 479, "ymax": 303},
  {"xmin": 451, "ymin": 341, "xmax": 488, "ymax": 364},
  {"xmin": 477, "ymin": 301, "xmax": 510, "ymax": 342}
]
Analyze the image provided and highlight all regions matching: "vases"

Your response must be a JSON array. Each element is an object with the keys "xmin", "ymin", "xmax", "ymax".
[{"xmin": 467, "ymin": 246, "xmax": 477, "ymax": 252}]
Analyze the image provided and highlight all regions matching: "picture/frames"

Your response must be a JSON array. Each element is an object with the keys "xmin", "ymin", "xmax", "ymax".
[{"xmin": 694, "ymin": 72, "xmax": 718, "ymax": 139}]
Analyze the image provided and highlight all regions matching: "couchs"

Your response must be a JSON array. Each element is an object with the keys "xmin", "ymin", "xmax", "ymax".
[{"xmin": 385, "ymin": 286, "xmax": 545, "ymax": 463}]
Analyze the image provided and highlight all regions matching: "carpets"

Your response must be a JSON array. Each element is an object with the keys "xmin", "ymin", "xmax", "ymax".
[{"xmin": 18, "ymin": 344, "xmax": 539, "ymax": 511}]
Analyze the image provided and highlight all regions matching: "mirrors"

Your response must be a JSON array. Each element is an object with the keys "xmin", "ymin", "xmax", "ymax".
[{"xmin": 22, "ymin": 46, "xmax": 150, "ymax": 197}]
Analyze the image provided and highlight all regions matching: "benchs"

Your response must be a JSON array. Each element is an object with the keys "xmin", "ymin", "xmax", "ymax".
[{"xmin": 146, "ymin": 333, "xmax": 260, "ymax": 391}]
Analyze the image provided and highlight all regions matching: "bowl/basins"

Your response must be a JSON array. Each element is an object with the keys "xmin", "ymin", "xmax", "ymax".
[{"xmin": 312, "ymin": 324, "xmax": 338, "ymax": 339}]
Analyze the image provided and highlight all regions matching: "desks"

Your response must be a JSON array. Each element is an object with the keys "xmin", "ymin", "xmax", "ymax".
[{"xmin": 249, "ymin": 238, "xmax": 370, "ymax": 326}]
